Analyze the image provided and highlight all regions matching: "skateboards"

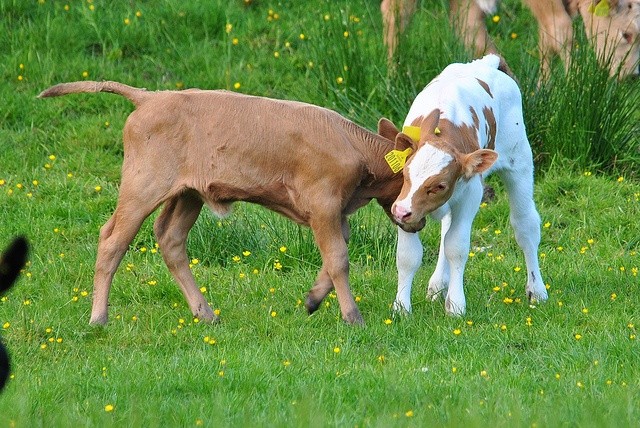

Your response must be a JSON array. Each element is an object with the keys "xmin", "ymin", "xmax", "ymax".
[
  {"xmin": 34, "ymin": 79, "xmax": 427, "ymax": 328},
  {"xmin": 380, "ymin": 0, "xmax": 640, "ymax": 92},
  {"xmin": 390, "ymin": 52, "xmax": 549, "ymax": 318}
]
